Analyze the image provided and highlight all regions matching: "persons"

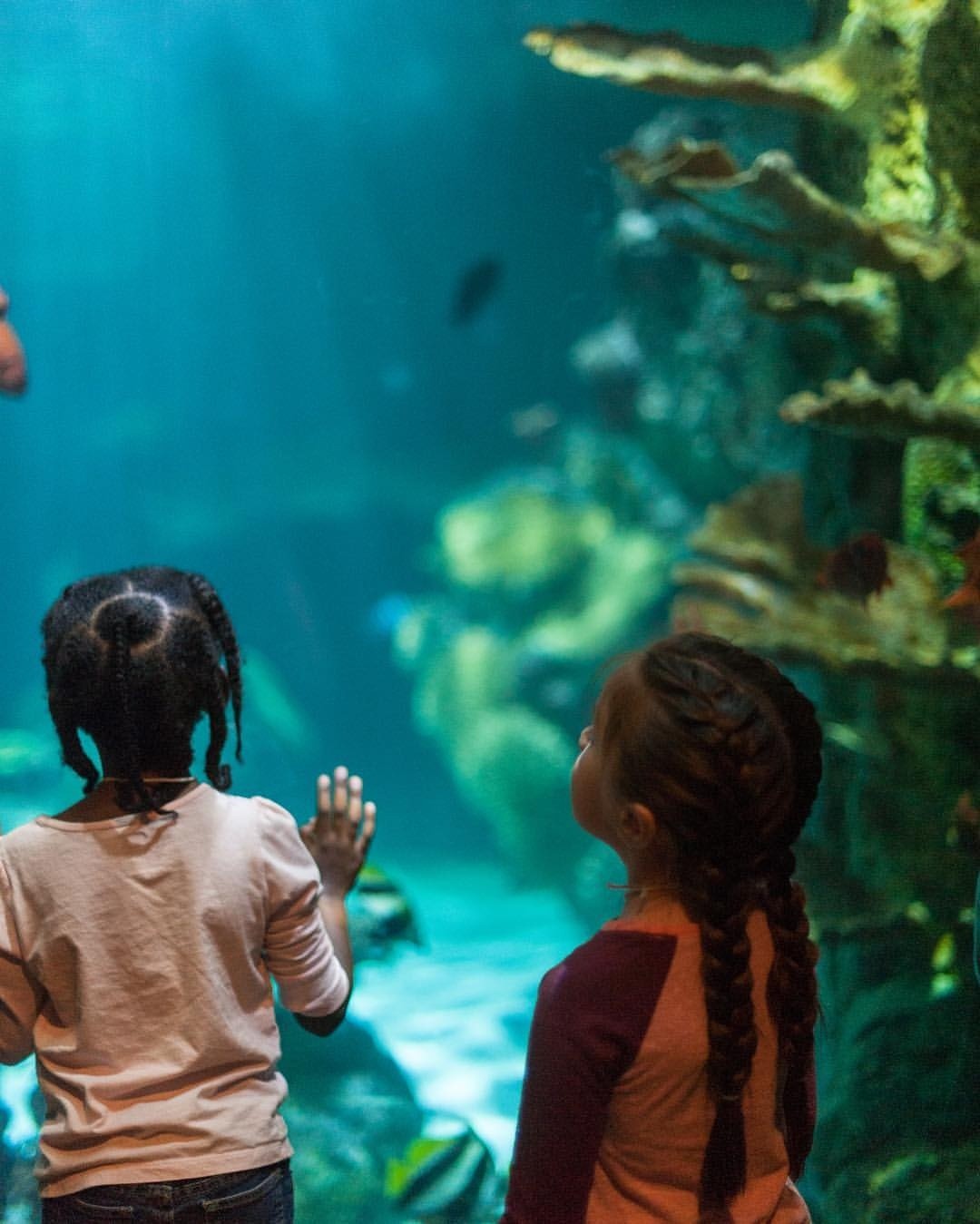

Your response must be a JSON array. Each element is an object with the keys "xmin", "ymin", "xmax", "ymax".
[
  {"xmin": 493, "ymin": 629, "xmax": 822, "ymax": 1224},
  {"xmin": 0, "ymin": 565, "xmax": 378, "ymax": 1224}
]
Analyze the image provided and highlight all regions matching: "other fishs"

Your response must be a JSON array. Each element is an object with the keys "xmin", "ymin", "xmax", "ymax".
[
  {"xmin": 352, "ymin": 866, "xmax": 421, "ymax": 960},
  {"xmin": 383, "ymin": 1124, "xmax": 509, "ymax": 1223}
]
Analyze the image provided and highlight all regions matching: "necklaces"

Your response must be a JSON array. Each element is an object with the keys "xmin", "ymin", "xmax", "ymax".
[
  {"xmin": 622, "ymin": 882, "xmax": 681, "ymax": 897},
  {"xmin": 99, "ymin": 775, "xmax": 198, "ymax": 781}
]
